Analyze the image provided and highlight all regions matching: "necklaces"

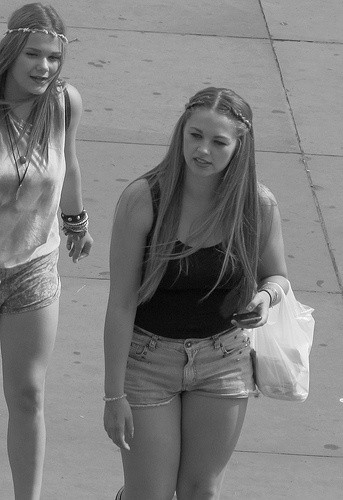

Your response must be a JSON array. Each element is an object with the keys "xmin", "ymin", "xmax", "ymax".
[
  {"xmin": 1, "ymin": 103, "xmax": 36, "ymax": 201},
  {"xmin": 7, "ymin": 110, "xmax": 31, "ymax": 164}
]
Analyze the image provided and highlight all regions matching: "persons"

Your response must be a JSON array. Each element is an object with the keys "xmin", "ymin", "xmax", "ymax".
[
  {"xmin": 0, "ymin": 2, "xmax": 94, "ymax": 500},
  {"xmin": 102, "ymin": 86, "xmax": 289, "ymax": 500}
]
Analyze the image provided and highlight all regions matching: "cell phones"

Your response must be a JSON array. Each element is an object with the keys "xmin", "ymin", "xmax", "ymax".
[{"xmin": 233, "ymin": 311, "xmax": 262, "ymax": 324}]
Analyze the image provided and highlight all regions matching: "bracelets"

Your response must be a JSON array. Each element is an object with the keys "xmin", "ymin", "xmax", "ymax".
[
  {"xmin": 61, "ymin": 209, "xmax": 88, "ymax": 239},
  {"xmin": 266, "ymin": 288, "xmax": 279, "ymax": 302},
  {"xmin": 103, "ymin": 393, "xmax": 128, "ymax": 401},
  {"xmin": 259, "ymin": 289, "xmax": 272, "ymax": 307}
]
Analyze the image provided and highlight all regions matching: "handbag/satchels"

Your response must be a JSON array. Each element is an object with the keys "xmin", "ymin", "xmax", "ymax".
[{"xmin": 248, "ymin": 275, "xmax": 315, "ymax": 402}]
